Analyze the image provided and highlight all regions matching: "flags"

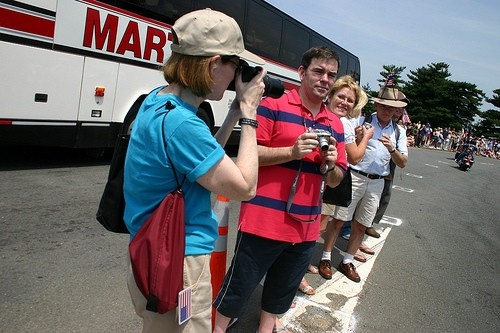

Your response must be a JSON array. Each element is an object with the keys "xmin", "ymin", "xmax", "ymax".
[
  {"xmin": 385, "ymin": 71, "xmax": 396, "ymax": 86},
  {"xmin": 179, "ymin": 288, "xmax": 191, "ymax": 323}
]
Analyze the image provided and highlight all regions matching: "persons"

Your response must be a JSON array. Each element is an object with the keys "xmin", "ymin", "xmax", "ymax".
[
  {"xmin": 340, "ymin": 71, "xmax": 410, "ymax": 262},
  {"xmin": 123, "ymin": 7, "xmax": 267, "ymax": 333},
  {"xmin": 211, "ymin": 45, "xmax": 347, "ymax": 333},
  {"xmin": 327, "ymin": 75, "xmax": 361, "ymax": 119},
  {"xmin": 298, "ymin": 90, "xmax": 375, "ymax": 295},
  {"xmin": 318, "ymin": 87, "xmax": 409, "ymax": 282},
  {"xmin": 406, "ymin": 121, "xmax": 500, "ymax": 168}
]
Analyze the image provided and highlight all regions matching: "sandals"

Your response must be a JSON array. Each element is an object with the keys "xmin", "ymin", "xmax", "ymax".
[
  {"xmin": 298, "ymin": 277, "xmax": 315, "ymax": 295},
  {"xmin": 273, "ymin": 328, "xmax": 292, "ymax": 333},
  {"xmin": 306, "ymin": 264, "xmax": 319, "ymax": 274}
]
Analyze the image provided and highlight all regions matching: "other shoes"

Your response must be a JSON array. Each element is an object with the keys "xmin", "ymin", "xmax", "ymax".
[
  {"xmin": 355, "ymin": 250, "xmax": 366, "ymax": 262},
  {"xmin": 359, "ymin": 243, "xmax": 375, "ymax": 255},
  {"xmin": 365, "ymin": 227, "xmax": 380, "ymax": 238},
  {"xmin": 342, "ymin": 234, "xmax": 350, "ymax": 240}
]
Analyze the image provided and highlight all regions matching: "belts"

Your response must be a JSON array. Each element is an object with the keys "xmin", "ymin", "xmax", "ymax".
[{"xmin": 350, "ymin": 167, "xmax": 385, "ymax": 180}]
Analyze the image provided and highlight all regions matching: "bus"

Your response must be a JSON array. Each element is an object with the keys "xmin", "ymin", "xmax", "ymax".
[{"xmin": 0, "ymin": 0, "xmax": 361, "ymax": 159}]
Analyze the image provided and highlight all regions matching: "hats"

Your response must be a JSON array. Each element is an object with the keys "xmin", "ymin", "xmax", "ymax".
[
  {"xmin": 171, "ymin": 8, "xmax": 267, "ymax": 66},
  {"xmin": 369, "ymin": 86, "xmax": 408, "ymax": 108},
  {"xmin": 398, "ymin": 91, "xmax": 410, "ymax": 103}
]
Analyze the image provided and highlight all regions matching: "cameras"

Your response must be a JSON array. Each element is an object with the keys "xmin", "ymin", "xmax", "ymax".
[
  {"xmin": 226, "ymin": 59, "xmax": 285, "ymax": 99},
  {"xmin": 308, "ymin": 129, "xmax": 331, "ymax": 151}
]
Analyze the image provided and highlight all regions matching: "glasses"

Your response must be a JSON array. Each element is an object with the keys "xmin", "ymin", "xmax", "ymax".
[
  {"xmin": 219, "ymin": 54, "xmax": 247, "ymax": 76},
  {"xmin": 377, "ymin": 103, "xmax": 397, "ymax": 111}
]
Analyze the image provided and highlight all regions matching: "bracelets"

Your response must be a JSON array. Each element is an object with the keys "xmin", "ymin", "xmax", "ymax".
[
  {"xmin": 238, "ymin": 117, "xmax": 258, "ymax": 129},
  {"xmin": 389, "ymin": 148, "xmax": 396, "ymax": 153}
]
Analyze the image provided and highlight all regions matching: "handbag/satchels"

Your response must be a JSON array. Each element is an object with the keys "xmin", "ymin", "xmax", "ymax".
[
  {"xmin": 96, "ymin": 93, "xmax": 215, "ymax": 234},
  {"xmin": 128, "ymin": 100, "xmax": 185, "ymax": 314},
  {"xmin": 322, "ymin": 167, "xmax": 352, "ymax": 207}
]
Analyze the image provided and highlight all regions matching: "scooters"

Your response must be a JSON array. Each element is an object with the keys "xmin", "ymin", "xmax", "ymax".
[{"xmin": 455, "ymin": 138, "xmax": 476, "ymax": 172}]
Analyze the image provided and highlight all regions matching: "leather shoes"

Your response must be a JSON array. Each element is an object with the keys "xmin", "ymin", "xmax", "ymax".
[
  {"xmin": 319, "ymin": 258, "xmax": 332, "ymax": 279},
  {"xmin": 337, "ymin": 259, "xmax": 360, "ymax": 282}
]
